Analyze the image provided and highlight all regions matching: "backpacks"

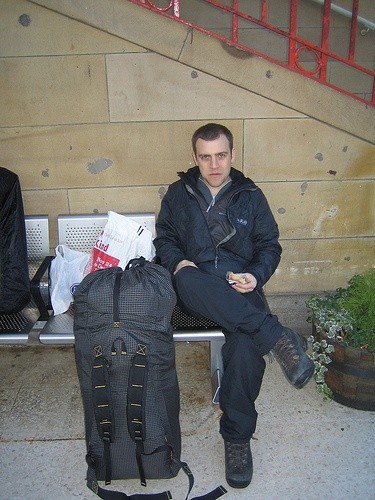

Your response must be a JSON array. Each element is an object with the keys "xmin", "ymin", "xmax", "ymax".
[{"xmin": 69, "ymin": 256, "xmax": 228, "ymax": 500}]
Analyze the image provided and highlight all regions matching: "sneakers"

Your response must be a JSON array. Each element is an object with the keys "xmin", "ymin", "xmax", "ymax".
[
  {"xmin": 224, "ymin": 437, "xmax": 253, "ymax": 488},
  {"xmin": 268, "ymin": 328, "xmax": 315, "ymax": 389}
]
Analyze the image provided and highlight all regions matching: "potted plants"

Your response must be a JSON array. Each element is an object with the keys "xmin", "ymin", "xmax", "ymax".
[{"xmin": 304, "ymin": 269, "xmax": 375, "ymax": 411}]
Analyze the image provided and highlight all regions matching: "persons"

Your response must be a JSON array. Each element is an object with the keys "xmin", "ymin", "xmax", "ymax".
[
  {"xmin": 150, "ymin": 122, "xmax": 316, "ymax": 490},
  {"xmin": 0, "ymin": 165, "xmax": 32, "ymax": 315}
]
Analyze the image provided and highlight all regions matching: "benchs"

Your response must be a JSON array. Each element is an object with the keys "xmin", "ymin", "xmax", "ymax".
[{"xmin": 0, "ymin": 212, "xmax": 228, "ymax": 406}]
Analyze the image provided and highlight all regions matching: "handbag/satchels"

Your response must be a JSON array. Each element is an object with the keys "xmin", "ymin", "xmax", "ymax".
[
  {"xmin": 48, "ymin": 245, "xmax": 93, "ymax": 315},
  {"xmin": 88, "ymin": 210, "xmax": 159, "ymax": 273}
]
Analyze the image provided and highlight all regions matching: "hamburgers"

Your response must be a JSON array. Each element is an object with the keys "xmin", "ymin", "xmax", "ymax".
[{"xmin": 227, "ymin": 271, "xmax": 246, "ymax": 284}]
